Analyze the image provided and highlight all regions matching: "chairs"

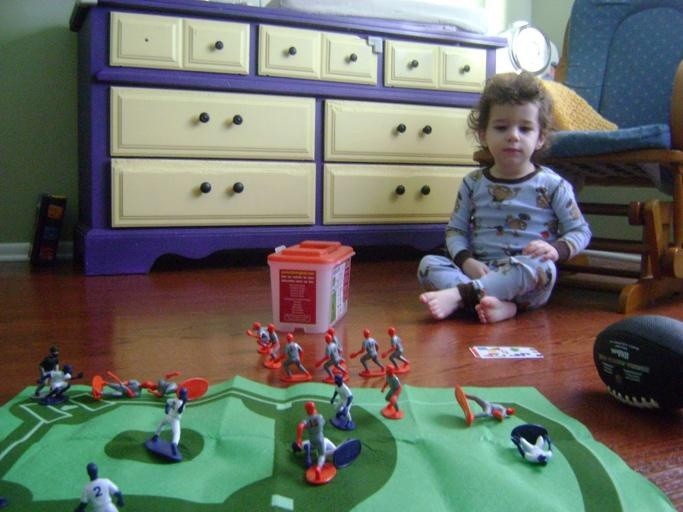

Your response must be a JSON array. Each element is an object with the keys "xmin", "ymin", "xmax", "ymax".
[{"xmin": 463, "ymin": 1, "xmax": 683, "ymax": 315}]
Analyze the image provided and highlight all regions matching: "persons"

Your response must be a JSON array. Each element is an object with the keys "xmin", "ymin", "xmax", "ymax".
[
  {"xmin": 380, "ymin": 365, "xmax": 401, "ymax": 418},
  {"xmin": 330, "ymin": 375, "xmax": 354, "ymax": 428},
  {"xmin": 152, "ymin": 388, "xmax": 188, "ymax": 456},
  {"xmin": 96, "ymin": 371, "xmax": 180, "ymax": 397},
  {"xmin": 414, "ymin": 69, "xmax": 592, "ymax": 324},
  {"xmin": 293, "ymin": 401, "xmax": 337, "ymax": 481},
  {"xmin": 465, "ymin": 393, "xmax": 514, "ymax": 420},
  {"xmin": 75, "ymin": 463, "xmax": 124, "ymax": 512},
  {"xmin": 247, "ymin": 322, "xmax": 407, "ymax": 379},
  {"xmin": 36, "ymin": 345, "xmax": 83, "ymax": 402},
  {"xmin": 512, "ymin": 430, "xmax": 552, "ymax": 465}
]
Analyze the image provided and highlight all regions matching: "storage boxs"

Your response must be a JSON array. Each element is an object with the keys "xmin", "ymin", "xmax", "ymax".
[{"xmin": 266, "ymin": 240, "xmax": 356, "ymax": 337}]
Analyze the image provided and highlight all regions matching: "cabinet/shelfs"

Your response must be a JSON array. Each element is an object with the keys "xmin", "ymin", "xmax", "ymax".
[{"xmin": 61, "ymin": 0, "xmax": 508, "ymax": 287}]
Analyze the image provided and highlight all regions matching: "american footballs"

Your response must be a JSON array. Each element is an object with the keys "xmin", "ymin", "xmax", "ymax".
[{"xmin": 595, "ymin": 314, "xmax": 682, "ymax": 409}]
